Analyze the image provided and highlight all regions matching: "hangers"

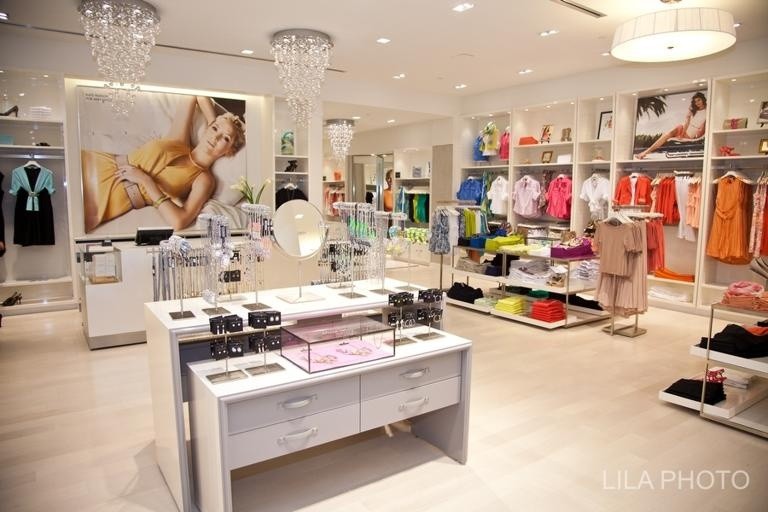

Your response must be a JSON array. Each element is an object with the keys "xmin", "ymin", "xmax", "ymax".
[
  {"xmin": 12, "ymin": 152, "xmax": 54, "ymax": 175},
  {"xmin": 284, "ymin": 177, "xmax": 297, "ymax": 190}
]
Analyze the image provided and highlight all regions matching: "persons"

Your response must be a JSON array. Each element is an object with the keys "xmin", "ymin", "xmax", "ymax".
[
  {"xmin": 635, "ymin": 92, "xmax": 706, "ymax": 161},
  {"xmin": 359, "ymin": 169, "xmax": 384, "ymax": 212},
  {"xmin": 81, "ymin": 95, "xmax": 245, "ymax": 233}
]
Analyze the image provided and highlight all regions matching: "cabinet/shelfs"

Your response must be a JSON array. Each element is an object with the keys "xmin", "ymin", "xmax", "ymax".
[
  {"xmin": 393, "ymin": 144, "xmax": 453, "ymax": 233},
  {"xmin": 0, "ymin": 116, "xmax": 65, "ymax": 151},
  {"xmin": 658, "ymin": 302, "xmax": 768, "ymax": 440},
  {"xmin": 275, "ymin": 155, "xmax": 309, "ymax": 175},
  {"xmin": 446, "ymin": 242, "xmax": 612, "ymax": 330},
  {"xmin": 185, "ymin": 319, "xmax": 474, "ymax": 512}
]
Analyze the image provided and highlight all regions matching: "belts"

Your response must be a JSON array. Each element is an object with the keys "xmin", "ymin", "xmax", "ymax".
[{"xmin": 116, "ymin": 155, "xmax": 145, "ymax": 208}]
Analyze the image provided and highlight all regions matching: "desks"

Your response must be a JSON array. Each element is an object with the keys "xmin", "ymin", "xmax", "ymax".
[{"xmin": 144, "ymin": 276, "xmax": 447, "ymax": 512}]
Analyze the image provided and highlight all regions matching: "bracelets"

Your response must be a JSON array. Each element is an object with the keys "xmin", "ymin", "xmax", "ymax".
[{"xmin": 153, "ymin": 195, "xmax": 169, "ymax": 209}]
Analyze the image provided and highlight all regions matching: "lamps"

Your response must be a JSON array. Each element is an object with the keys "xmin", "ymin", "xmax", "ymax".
[
  {"xmin": 77, "ymin": 0, "xmax": 162, "ymax": 118},
  {"xmin": 270, "ymin": 28, "xmax": 335, "ymax": 130},
  {"xmin": 325, "ymin": 119, "xmax": 356, "ymax": 163},
  {"xmin": 610, "ymin": 7, "xmax": 737, "ymax": 63}
]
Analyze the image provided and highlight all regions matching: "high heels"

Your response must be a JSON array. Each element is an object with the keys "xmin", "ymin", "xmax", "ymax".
[
  {"xmin": 719, "ymin": 146, "xmax": 738, "ymax": 155},
  {"xmin": 0, "ymin": 292, "xmax": 23, "ymax": 306}
]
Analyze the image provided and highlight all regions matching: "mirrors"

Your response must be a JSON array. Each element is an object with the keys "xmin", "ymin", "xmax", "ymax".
[{"xmin": 271, "ymin": 199, "xmax": 326, "ymax": 259}]
